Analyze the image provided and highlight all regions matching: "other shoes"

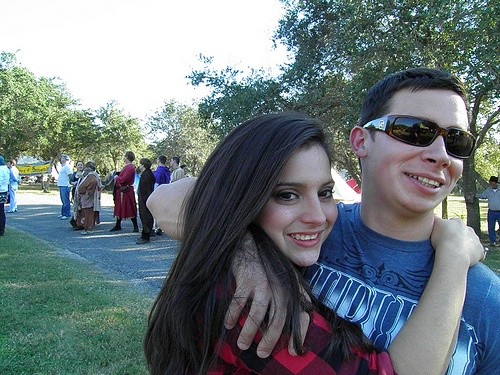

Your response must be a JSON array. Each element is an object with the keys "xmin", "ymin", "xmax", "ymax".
[
  {"xmin": 133, "ymin": 229, "xmax": 139, "ymax": 233},
  {"xmin": 61, "ymin": 216, "xmax": 67, "ymax": 220},
  {"xmin": 151, "ymin": 231, "xmax": 155, "ymax": 236},
  {"xmin": 156, "ymin": 230, "xmax": 162, "ymax": 235},
  {"xmin": 81, "ymin": 230, "xmax": 93, "ymax": 234},
  {"xmin": 136, "ymin": 239, "xmax": 148, "ymax": 244},
  {"xmin": 110, "ymin": 226, "xmax": 121, "ymax": 231},
  {"xmin": 6, "ymin": 210, "xmax": 14, "ymax": 213}
]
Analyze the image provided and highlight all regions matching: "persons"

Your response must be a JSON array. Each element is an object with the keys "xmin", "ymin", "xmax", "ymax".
[
  {"xmin": 143, "ymin": 112, "xmax": 484, "ymax": 375},
  {"xmin": 146, "ymin": 67, "xmax": 500, "ymax": 375},
  {"xmin": 110, "ymin": 151, "xmax": 139, "ymax": 233},
  {"xmin": 69, "ymin": 161, "xmax": 105, "ymax": 234},
  {"xmin": 57, "ymin": 155, "xmax": 73, "ymax": 220},
  {"xmin": 154, "ymin": 155, "xmax": 170, "ymax": 235},
  {"xmin": 6, "ymin": 158, "xmax": 19, "ymax": 213},
  {"xmin": 134, "ymin": 158, "xmax": 155, "ymax": 244},
  {"xmin": 170, "ymin": 157, "xmax": 189, "ymax": 184},
  {"xmin": 0, "ymin": 155, "xmax": 10, "ymax": 236},
  {"xmin": 474, "ymin": 176, "xmax": 500, "ymax": 247}
]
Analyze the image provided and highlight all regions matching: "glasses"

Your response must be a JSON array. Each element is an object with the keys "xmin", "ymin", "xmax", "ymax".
[{"xmin": 363, "ymin": 115, "xmax": 475, "ymax": 159}]
